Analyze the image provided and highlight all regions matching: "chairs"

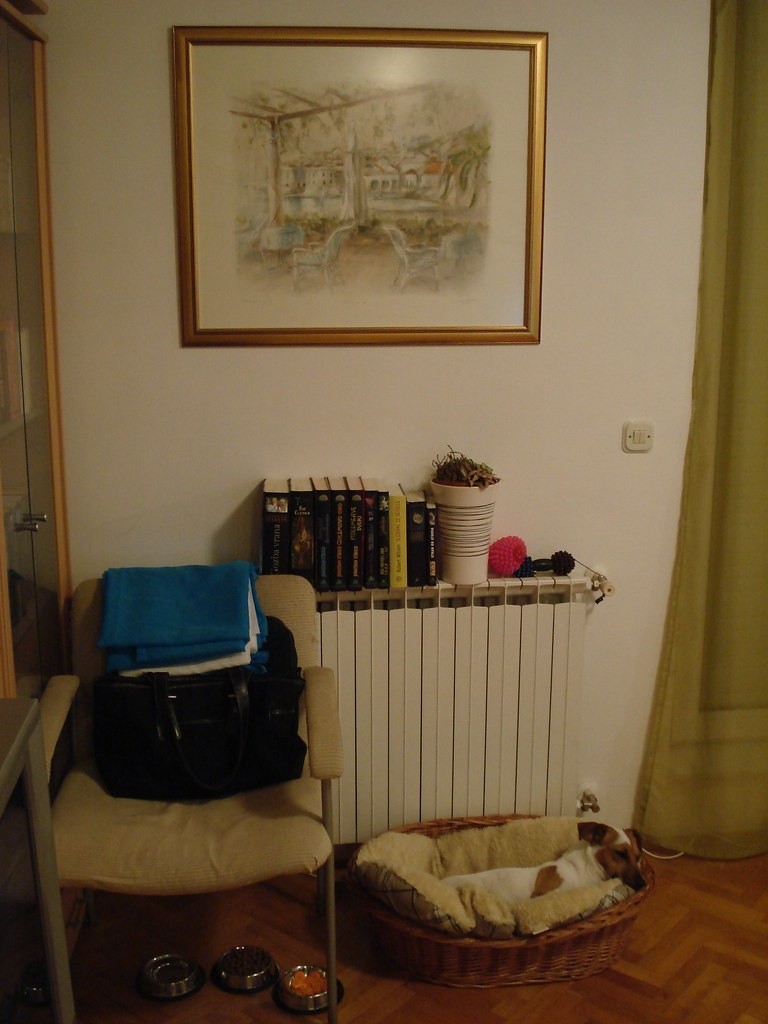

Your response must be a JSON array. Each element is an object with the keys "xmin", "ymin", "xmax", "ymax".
[{"xmin": 40, "ymin": 576, "xmax": 343, "ymax": 1024}]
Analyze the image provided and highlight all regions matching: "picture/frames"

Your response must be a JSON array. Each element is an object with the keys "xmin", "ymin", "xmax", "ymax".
[{"xmin": 171, "ymin": 24, "xmax": 550, "ymax": 349}]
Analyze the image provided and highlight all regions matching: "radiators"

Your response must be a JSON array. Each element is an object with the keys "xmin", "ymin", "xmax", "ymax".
[{"xmin": 315, "ymin": 570, "xmax": 615, "ymax": 846}]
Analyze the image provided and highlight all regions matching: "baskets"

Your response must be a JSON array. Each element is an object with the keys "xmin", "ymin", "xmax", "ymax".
[{"xmin": 349, "ymin": 813, "xmax": 655, "ymax": 988}]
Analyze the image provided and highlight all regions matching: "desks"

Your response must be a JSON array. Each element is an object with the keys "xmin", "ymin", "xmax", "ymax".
[{"xmin": 0, "ymin": 697, "xmax": 77, "ymax": 1024}]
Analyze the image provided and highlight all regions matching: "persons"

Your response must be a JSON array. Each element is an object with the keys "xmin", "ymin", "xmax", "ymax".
[
  {"xmin": 277, "ymin": 499, "xmax": 288, "ymax": 513},
  {"xmin": 267, "ymin": 497, "xmax": 277, "ymax": 512}
]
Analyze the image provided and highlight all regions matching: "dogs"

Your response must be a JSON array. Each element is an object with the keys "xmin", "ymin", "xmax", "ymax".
[{"xmin": 438, "ymin": 820, "xmax": 647, "ymax": 905}]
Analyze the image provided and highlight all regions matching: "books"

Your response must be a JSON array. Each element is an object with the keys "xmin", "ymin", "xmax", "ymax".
[{"xmin": 262, "ymin": 476, "xmax": 437, "ymax": 592}]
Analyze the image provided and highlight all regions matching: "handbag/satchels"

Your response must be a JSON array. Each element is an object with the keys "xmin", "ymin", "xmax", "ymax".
[{"xmin": 93, "ymin": 665, "xmax": 307, "ymax": 803}]
